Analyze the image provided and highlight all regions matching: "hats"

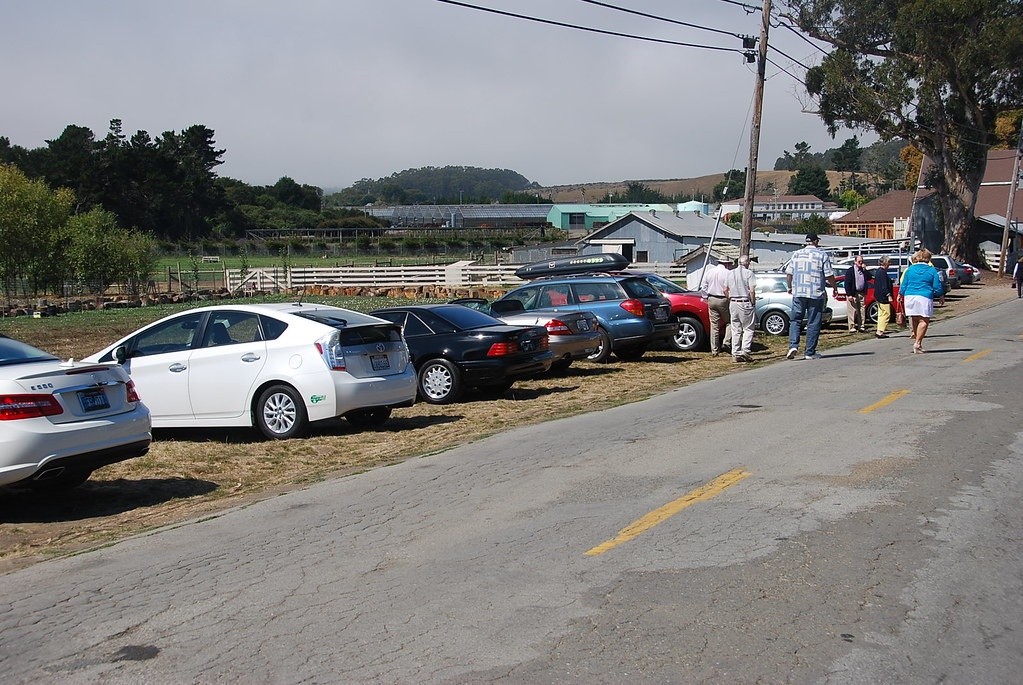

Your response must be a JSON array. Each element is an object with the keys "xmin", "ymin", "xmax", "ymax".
[
  {"xmin": 806, "ymin": 233, "xmax": 821, "ymax": 242},
  {"xmin": 715, "ymin": 255, "xmax": 733, "ymax": 264}
]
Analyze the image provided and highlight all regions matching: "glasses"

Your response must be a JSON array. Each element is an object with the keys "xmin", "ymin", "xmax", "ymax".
[{"xmin": 857, "ymin": 261, "xmax": 863, "ymax": 263}]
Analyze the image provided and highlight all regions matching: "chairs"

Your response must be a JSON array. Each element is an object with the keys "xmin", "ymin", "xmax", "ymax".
[
  {"xmin": 589, "ymin": 292, "xmax": 599, "ymax": 300},
  {"xmin": 212, "ymin": 323, "xmax": 231, "ymax": 345}
]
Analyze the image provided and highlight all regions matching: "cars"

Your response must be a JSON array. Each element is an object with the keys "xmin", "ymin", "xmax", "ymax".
[
  {"xmin": 961, "ymin": 263, "xmax": 981, "ymax": 282},
  {"xmin": 79, "ymin": 301, "xmax": 418, "ymax": 440},
  {"xmin": 930, "ymin": 253, "xmax": 961, "ymax": 288},
  {"xmin": 0, "ymin": 332, "xmax": 152, "ymax": 491},
  {"xmin": 369, "ymin": 303, "xmax": 552, "ymax": 405},
  {"xmin": 951, "ymin": 262, "xmax": 975, "ymax": 288},
  {"xmin": 753, "ymin": 244, "xmax": 951, "ymax": 336},
  {"xmin": 615, "ymin": 270, "xmax": 728, "ymax": 353},
  {"xmin": 446, "ymin": 298, "xmax": 603, "ymax": 380}
]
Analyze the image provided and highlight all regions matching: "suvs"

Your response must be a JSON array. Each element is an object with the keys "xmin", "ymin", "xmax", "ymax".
[{"xmin": 493, "ymin": 253, "xmax": 680, "ymax": 363}]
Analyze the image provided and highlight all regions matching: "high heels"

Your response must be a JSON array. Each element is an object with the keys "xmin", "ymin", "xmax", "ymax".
[{"xmin": 912, "ymin": 343, "xmax": 926, "ymax": 354}]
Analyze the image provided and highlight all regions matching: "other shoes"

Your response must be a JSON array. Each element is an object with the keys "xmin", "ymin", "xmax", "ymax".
[
  {"xmin": 731, "ymin": 356, "xmax": 739, "ymax": 362},
  {"xmin": 721, "ymin": 344, "xmax": 731, "ymax": 354},
  {"xmin": 804, "ymin": 353, "xmax": 821, "ymax": 360},
  {"xmin": 850, "ymin": 328, "xmax": 857, "ymax": 333},
  {"xmin": 712, "ymin": 349, "xmax": 720, "ymax": 357},
  {"xmin": 741, "ymin": 351, "xmax": 754, "ymax": 362},
  {"xmin": 787, "ymin": 348, "xmax": 798, "ymax": 359},
  {"xmin": 875, "ymin": 333, "xmax": 889, "ymax": 338},
  {"xmin": 860, "ymin": 325, "xmax": 865, "ymax": 331},
  {"xmin": 1018, "ymin": 296, "xmax": 1021, "ymax": 298}
]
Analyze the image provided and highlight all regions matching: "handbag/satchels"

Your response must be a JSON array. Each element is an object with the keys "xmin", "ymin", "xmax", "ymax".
[
  {"xmin": 896, "ymin": 301, "xmax": 906, "ymax": 327},
  {"xmin": 1011, "ymin": 282, "xmax": 1016, "ymax": 288}
]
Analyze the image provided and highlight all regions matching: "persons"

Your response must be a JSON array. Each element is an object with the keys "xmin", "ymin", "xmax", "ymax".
[
  {"xmin": 1013, "ymin": 257, "xmax": 1023, "ymax": 298},
  {"xmin": 787, "ymin": 234, "xmax": 838, "ymax": 360},
  {"xmin": 897, "ymin": 248, "xmax": 945, "ymax": 353},
  {"xmin": 723, "ymin": 255, "xmax": 757, "ymax": 363},
  {"xmin": 844, "ymin": 255, "xmax": 872, "ymax": 332},
  {"xmin": 701, "ymin": 255, "xmax": 733, "ymax": 357},
  {"xmin": 874, "ymin": 255, "xmax": 894, "ymax": 338}
]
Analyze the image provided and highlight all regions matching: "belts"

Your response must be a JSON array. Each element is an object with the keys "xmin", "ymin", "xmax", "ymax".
[
  {"xmin": 709, "ymin": 294, "xmax": 725, "ymax": 298},
  {"xmin": 731, "ymin": 300, "xmax": 749, "ymax": 302}
]
